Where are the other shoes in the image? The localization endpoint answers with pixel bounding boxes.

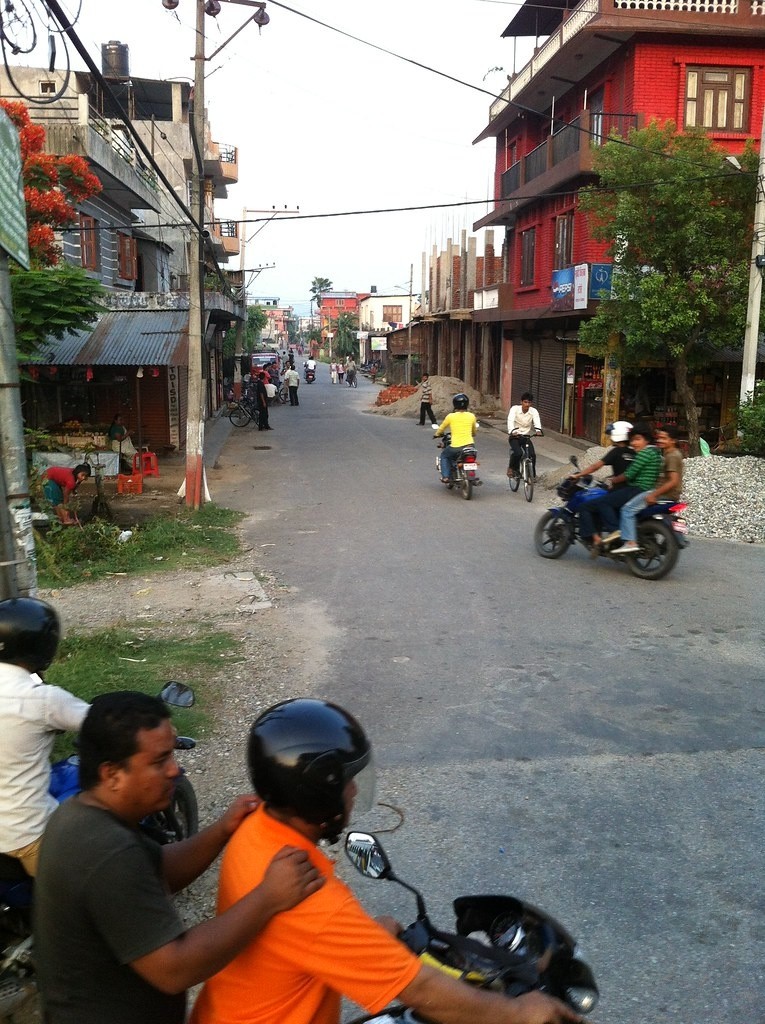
[590,538,602,559]
[610,542,642,553]
[291,403,295,406]
[295,402,299,406]
[63,519,79,525]
[529,475,537,483]
[416,422,424,426]
[265,425,274,430]
[602,531,620,543]
[507,467,514,478]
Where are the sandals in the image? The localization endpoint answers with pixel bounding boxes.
[439,476,449,483]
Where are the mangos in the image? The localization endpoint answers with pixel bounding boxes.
[64,420,80,426]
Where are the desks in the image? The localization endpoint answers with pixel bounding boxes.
[32,449,120,477]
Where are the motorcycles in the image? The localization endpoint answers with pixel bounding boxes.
[431,421,485,501]
[533,455,691,580]
[0,679,199,1022]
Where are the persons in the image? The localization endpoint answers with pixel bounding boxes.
[304,356,317,381]
[345,354,356,387]
[108,412,141,469]
[369,364,377,384]
[0,597,91,880]
[506,391,543,483]
[337,358,346,383]
[256,372,275,431]
[330,358,339,384]
[41,463,92,525]
[185,699,585,1024]
[298,345,305,357]
[271,348,294,369]
[568,422,684,554]
[433,393,477,482]
[32,690,328,1024]
[261,360,300,406]
[415,374,437,426]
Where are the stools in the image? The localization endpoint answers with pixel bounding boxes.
[132,443,150,452]
[133,452,159,478]
[116,474,143,494]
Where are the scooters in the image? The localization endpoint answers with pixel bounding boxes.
[345,370,359,387]
[345,829,604,1024]
[303,362,318,383]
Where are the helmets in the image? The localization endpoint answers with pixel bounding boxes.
[605,420,634,442]
[309,356,314,359]
[0,597,60,672]
[453,394,469,409]
[247,697,375,829]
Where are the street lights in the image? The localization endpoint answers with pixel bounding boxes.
[163,0,270,517]
[395,286,412,386]
[322,305,333,358]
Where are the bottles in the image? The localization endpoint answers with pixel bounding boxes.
[655,406,677,425]
[585,364,604,379]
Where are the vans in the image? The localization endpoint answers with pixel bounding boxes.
[251,353,280,381]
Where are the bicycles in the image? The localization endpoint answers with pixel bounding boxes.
[229,388,266,429]
[508,425,545,502]
[272,381,291,403]
